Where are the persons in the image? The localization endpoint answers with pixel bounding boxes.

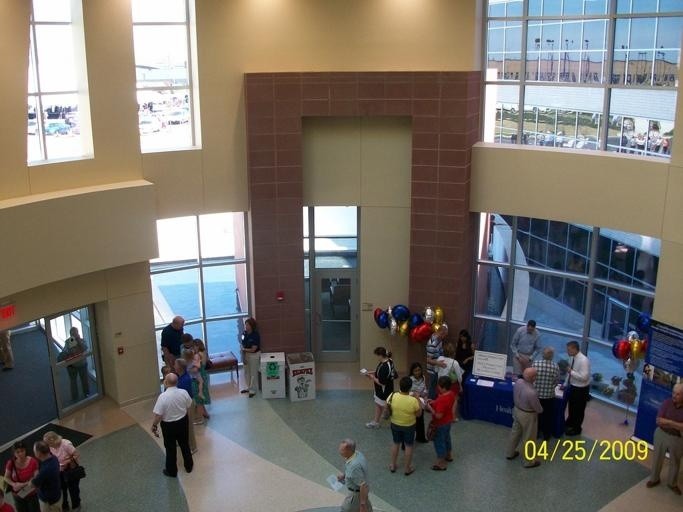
[646,384,683,497]
[149,316,211,477]
[360,326,477,474]
[508,319,590,469]
[54,327,89,400]
[336,439,374,511]
[1,430,81,512]
[236,317,260,396]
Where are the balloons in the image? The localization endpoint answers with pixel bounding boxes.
[371,303,449,343]
[613,330,647,422]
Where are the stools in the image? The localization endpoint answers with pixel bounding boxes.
[208,351,239,385]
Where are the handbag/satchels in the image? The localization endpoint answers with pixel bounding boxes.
[205,351,213,371]
[449,360,458,381]
[56,348,72,368]
[63,458,86,484]
[383,392,395,419]
[393,361,399,379]
[427,411,438,441]
[3,459,15,494]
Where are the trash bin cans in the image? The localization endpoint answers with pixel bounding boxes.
[260,352,286,399]
[287,352,315,401]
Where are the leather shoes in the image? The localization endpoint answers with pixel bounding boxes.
[524,460,540,468]
[249,393,254,398]
[241,390,249,393]
[505,451,519,460]
[647,479,660,488]
[163,469,177,478]
[667,484,681,495]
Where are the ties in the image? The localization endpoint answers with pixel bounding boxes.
[568,357,575,387]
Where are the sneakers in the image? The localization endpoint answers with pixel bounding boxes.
[191,449,197,455]
[193,421,204,425]
[366,421,380,429]
[415,439,429,443]
[71,506,81,512]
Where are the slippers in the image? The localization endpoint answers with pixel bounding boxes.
[430,464,448,472]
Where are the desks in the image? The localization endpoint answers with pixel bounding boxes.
[459,372,570,439]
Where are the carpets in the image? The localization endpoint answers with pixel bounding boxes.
[0,423,93,494]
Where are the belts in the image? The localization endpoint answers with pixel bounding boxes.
[660,427,681,437]
[349,488,360,492]
[571,386,590,390]
[515,406,536,413]
[519,351,533,355]
